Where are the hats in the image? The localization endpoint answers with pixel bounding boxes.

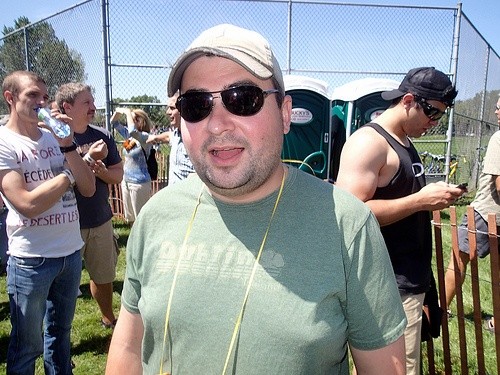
[381,67,458,108]
[166,24,285,99]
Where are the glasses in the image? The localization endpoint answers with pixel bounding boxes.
[174,83,279,123]
[414,95,445,121]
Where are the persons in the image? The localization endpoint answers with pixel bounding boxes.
[0,69,125,375]
[336,66,470,375]
[104,23,408,375]
[110,91,192,226]
[438,96,500,329]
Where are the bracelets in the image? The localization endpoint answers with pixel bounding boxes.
[84,153,96,166]
[62,169,76,187]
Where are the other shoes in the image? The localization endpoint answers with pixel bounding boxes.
[101,316,117,331]
[438,298,452,322]
[488,317,495,332]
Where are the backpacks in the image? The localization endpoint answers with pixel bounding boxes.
[141,145,158,181]
